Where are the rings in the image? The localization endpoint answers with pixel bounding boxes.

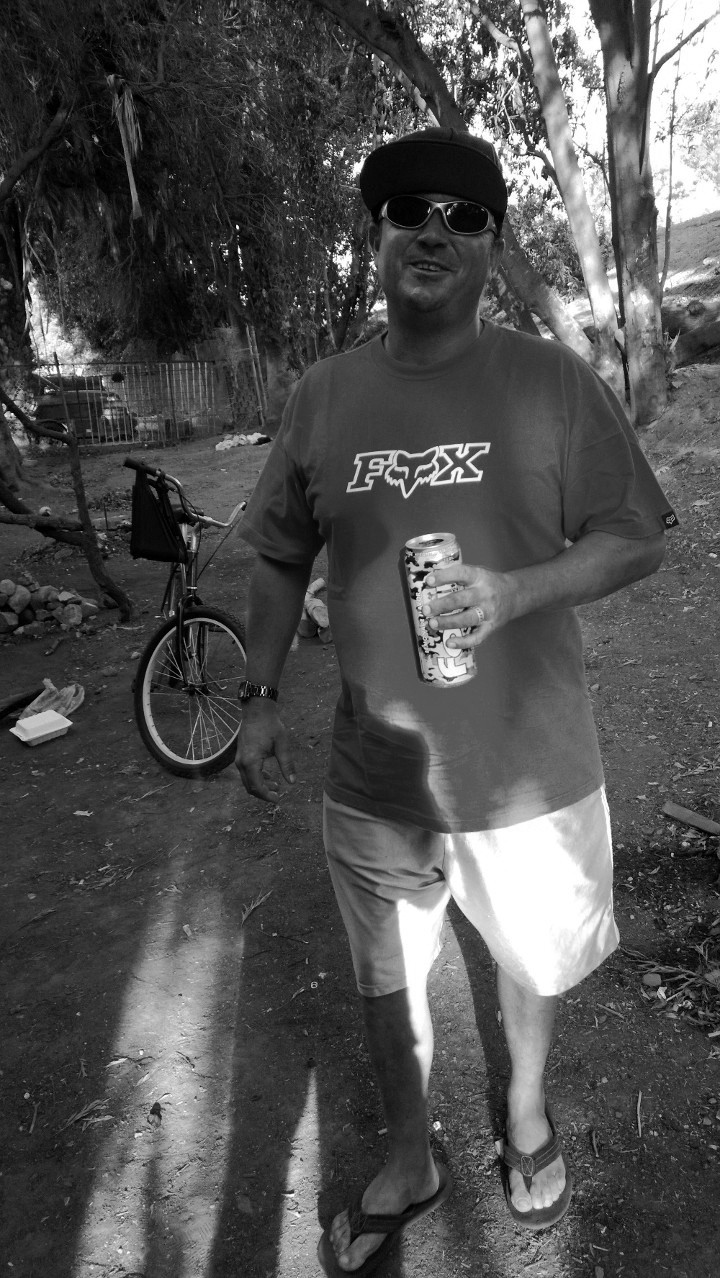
[470,606,485,626]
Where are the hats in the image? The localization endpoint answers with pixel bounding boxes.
[359,129,508,227]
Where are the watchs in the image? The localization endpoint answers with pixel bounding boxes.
[238,680,277,704]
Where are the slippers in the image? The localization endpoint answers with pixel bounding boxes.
[317,1164,453,1278]
[500,1097,573,1231]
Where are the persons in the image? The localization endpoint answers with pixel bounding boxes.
[245,126,683,1278]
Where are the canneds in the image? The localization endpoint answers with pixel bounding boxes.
[405,533,476,688]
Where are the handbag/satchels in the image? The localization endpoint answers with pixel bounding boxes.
[130,465,190,562]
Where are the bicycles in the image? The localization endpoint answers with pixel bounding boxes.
[123,453,260,780]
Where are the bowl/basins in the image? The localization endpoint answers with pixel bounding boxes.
[9,710,73,746]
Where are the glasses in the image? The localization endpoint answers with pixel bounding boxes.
[377,195,498,238]
